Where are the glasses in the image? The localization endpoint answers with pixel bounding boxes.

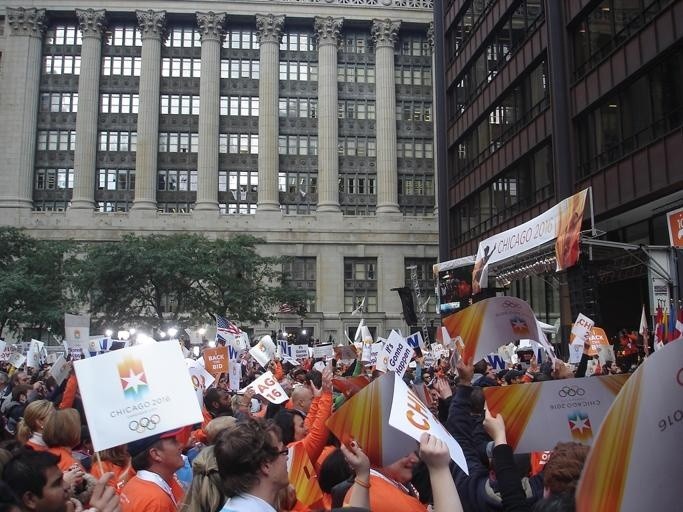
[276,448,289,455]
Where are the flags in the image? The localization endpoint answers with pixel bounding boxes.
[215,314,237,333]
[640,300,681,351]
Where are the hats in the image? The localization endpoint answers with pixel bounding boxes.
[127,429,183,456]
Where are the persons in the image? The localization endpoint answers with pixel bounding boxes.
[0,331,682,511]
[439,271,471,303]
[471,244,496,293]
